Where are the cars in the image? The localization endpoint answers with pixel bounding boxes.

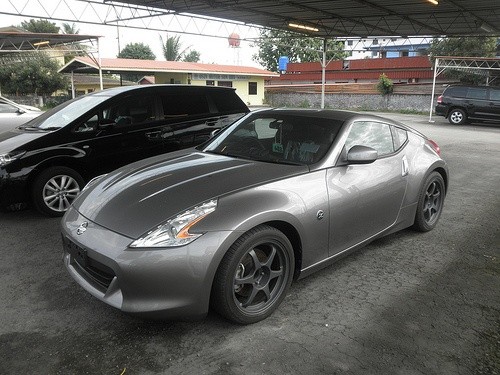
[0,95,47,135]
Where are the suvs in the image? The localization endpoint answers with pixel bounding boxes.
[434,83,500,126]
[0,81,259,219]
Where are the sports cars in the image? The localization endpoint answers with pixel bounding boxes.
[59,106,451,326]
